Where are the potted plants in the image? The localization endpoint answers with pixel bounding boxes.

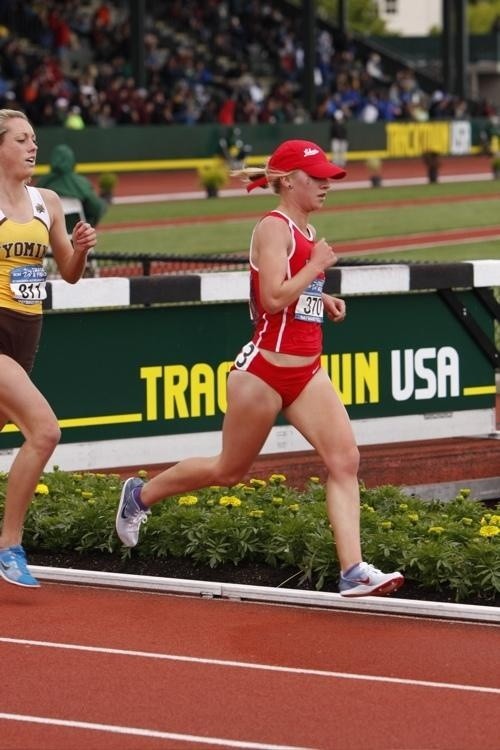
[199,165,228,196]
[98,172,117,203]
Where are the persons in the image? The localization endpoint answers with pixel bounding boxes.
[329,110,350,167]
[0,110,99,590]
[114,136,407,599]
[35,143,108,279]
[218,126,252,171]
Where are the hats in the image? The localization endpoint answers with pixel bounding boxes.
[264,138,348,181]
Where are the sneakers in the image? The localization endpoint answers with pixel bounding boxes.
[337,560,406,598]
[113,473,153,549]
[0,542,41,589]
